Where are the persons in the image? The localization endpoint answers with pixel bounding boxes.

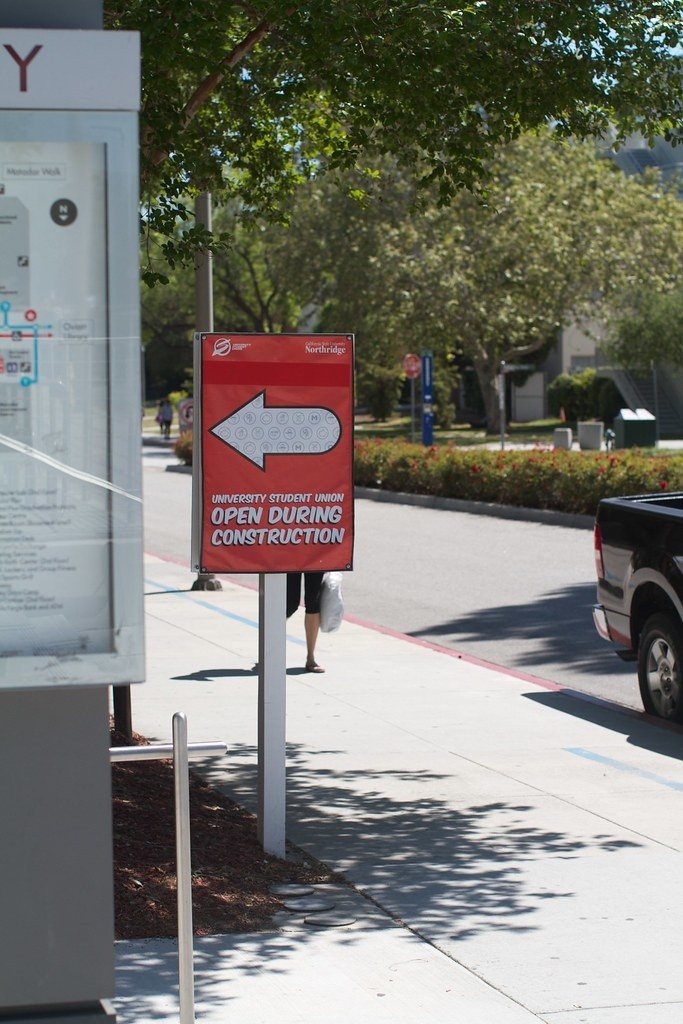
[286,571,327,674]
[155,398,173,440]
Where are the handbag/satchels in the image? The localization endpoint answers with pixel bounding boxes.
[319,570,344,632]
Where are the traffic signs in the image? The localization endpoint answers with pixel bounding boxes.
[191,330,356,578]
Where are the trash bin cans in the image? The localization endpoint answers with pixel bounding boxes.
[615,408,656,451]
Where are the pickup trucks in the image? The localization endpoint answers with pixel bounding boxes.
[590,490,683,726]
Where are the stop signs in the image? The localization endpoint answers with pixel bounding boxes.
[404,355,421,379]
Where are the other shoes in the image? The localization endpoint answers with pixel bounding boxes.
[306,662,324,672]
[252,662,259,672]
